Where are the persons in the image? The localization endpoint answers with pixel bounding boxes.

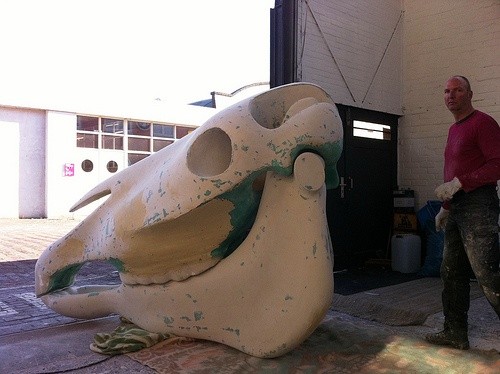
[426,76,500,350]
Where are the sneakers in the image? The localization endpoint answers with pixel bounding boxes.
[424,329,469,351]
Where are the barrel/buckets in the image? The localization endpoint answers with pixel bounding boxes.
[391,233,421,274]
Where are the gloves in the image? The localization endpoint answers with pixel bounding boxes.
[434,206,452,233]
[432,176,463,203]
